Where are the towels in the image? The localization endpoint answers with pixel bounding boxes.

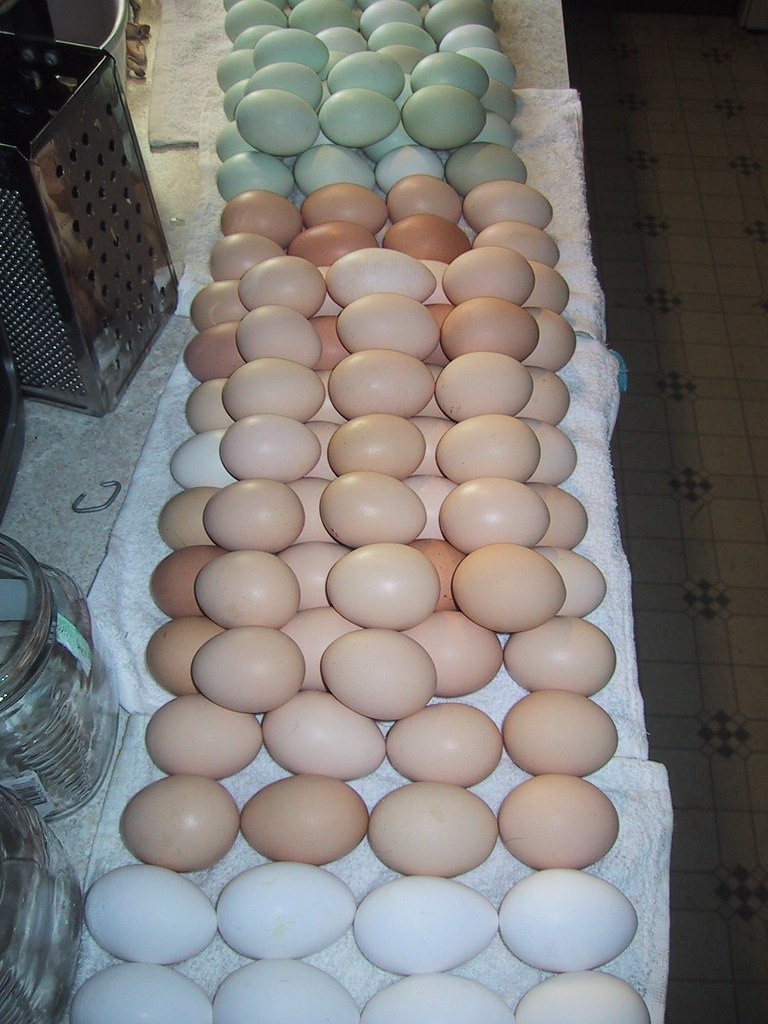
[57,2,677,1024]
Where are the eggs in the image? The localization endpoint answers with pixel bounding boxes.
[68,0,651,1024]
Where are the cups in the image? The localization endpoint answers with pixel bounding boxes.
[0,0,128,94]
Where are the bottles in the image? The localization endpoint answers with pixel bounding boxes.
[0,785,83,1024]
[0,533,118,821]
